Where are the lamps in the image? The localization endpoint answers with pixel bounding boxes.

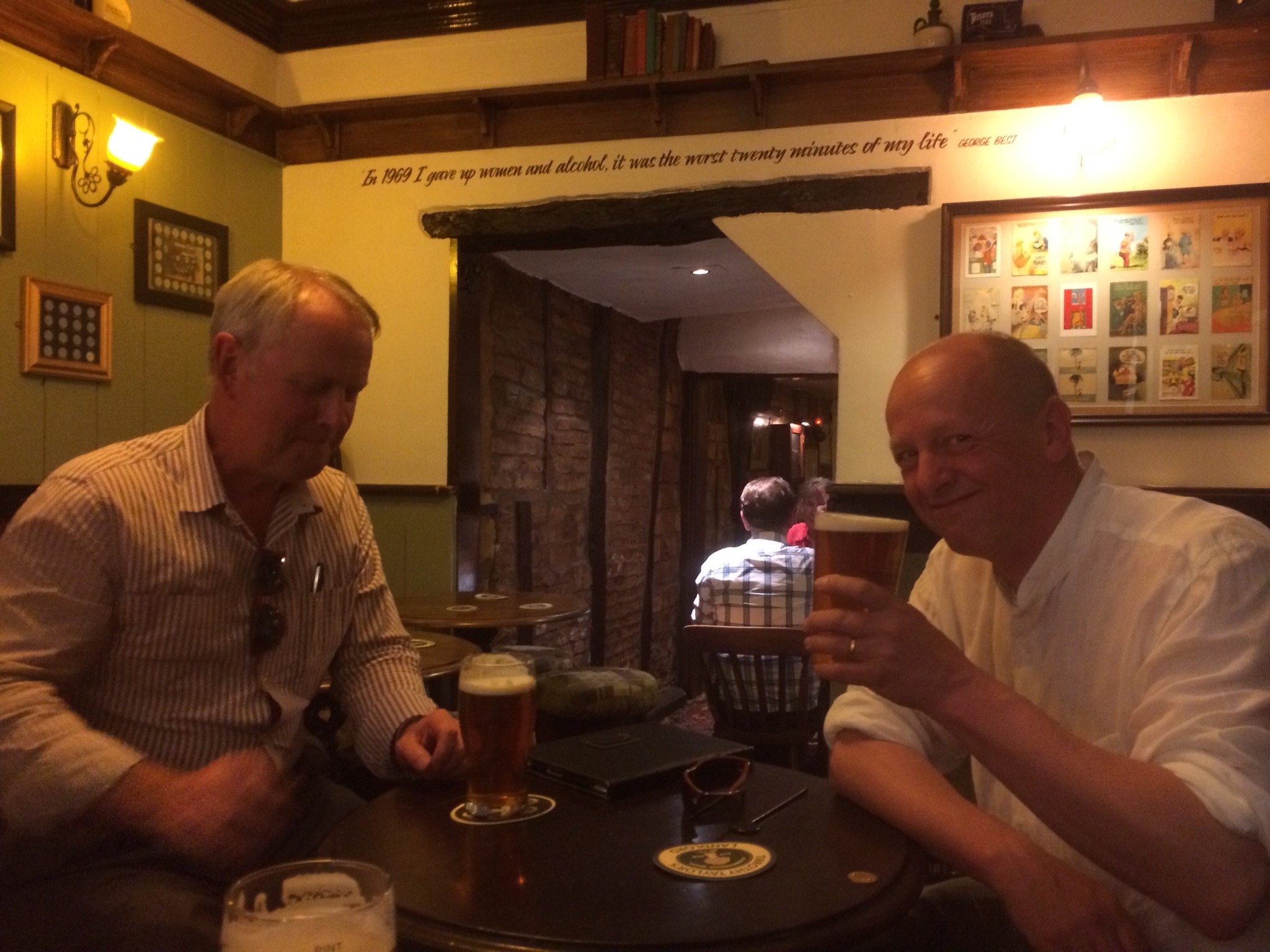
[1071,56,1105,105]
[51,100,168,211]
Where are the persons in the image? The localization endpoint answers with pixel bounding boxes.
[0,260,475,952]
[796,481,829,549]
[692,474,828,727]
[797,331,1270,952]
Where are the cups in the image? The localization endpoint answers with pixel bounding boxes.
[218,858,399,951]
[455,650,538,820]
[808,509,910,666]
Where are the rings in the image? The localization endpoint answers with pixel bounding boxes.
[849,638,856,655]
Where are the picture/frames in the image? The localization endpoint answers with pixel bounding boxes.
[17,275,115,384]
[131,198,229,313]
[0,98,18,254]
[935,182,1269,428]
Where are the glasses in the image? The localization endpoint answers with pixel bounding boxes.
[248,546,288,657]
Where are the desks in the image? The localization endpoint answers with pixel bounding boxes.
[304,633,482,762]
[682,624,830,773]
[390,591,588,652]
[316,758,925,952]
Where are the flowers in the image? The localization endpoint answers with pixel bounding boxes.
[786,523,809,548]
